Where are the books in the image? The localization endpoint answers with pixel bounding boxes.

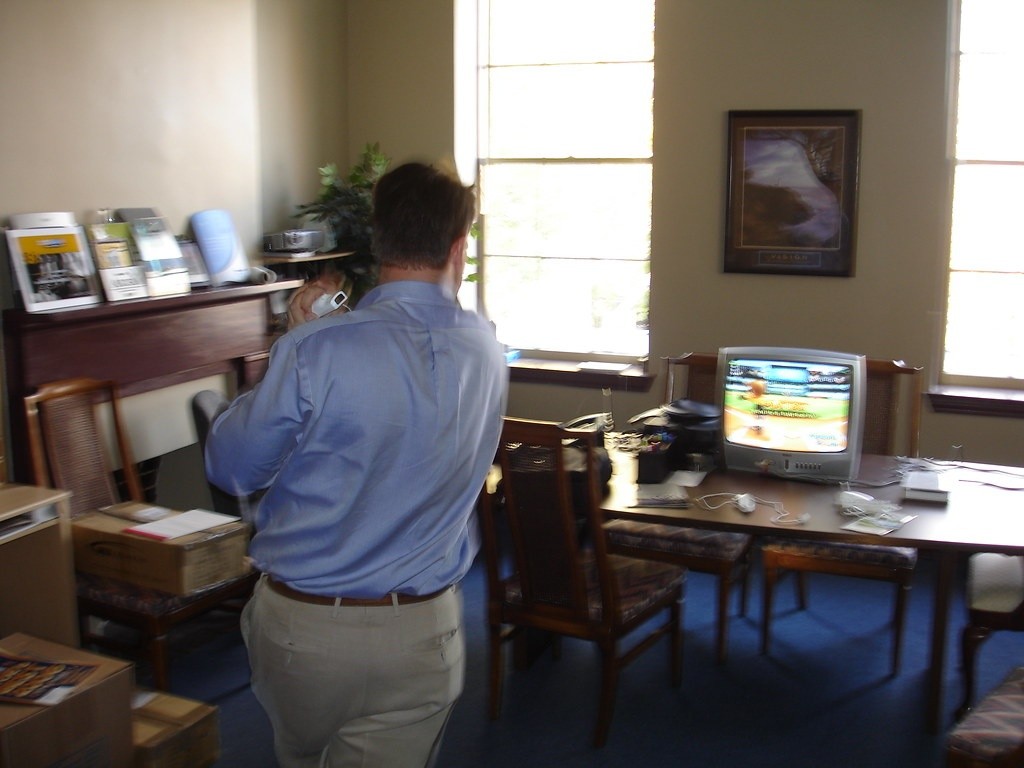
[906,470,951,503]
[627,483,690,508]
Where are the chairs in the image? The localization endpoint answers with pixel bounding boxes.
[952,551,1024,719]
[476,416,687,747]
[24,377,258,705]
[761,357,924,676]
[599,353,753,662]
[943,665,1024,768]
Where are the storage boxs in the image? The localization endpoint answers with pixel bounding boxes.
[132,685,223,768]
[0,632,133,768]
[70,500,251,598]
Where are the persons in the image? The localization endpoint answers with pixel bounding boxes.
[206,161,509,768]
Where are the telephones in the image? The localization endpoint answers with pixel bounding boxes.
[557,413,615,448]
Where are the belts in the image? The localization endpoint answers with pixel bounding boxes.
[266,574,454,606]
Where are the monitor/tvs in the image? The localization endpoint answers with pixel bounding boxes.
[713,347,867,483]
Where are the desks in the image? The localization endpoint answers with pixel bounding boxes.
[484,432,1024,552]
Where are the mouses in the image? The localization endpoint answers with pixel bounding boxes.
[734,494,757,513]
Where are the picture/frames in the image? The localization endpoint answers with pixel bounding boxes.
[5,226,103,312]
[722,108,862,281]
